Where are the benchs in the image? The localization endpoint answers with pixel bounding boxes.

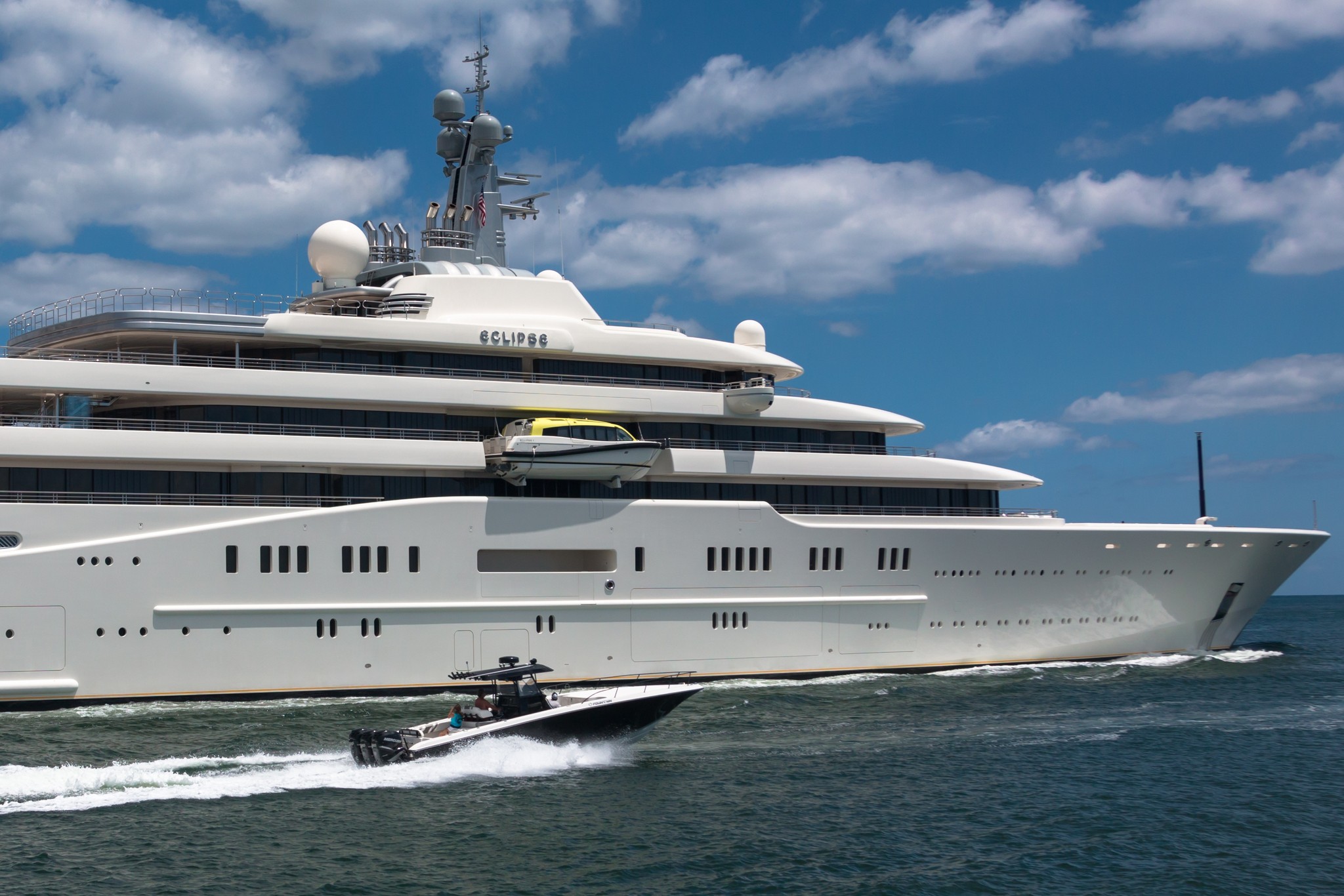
[446,706,498,735]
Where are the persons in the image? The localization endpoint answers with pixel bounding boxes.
[438,704,464,736]
[475,688,500,720]
[549,692,561,708]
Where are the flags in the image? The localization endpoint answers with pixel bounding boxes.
[477,182,486,228]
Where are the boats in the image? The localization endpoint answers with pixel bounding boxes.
[481,416,664,482]
[0,19,1331,708]
[349,656,705,768]
